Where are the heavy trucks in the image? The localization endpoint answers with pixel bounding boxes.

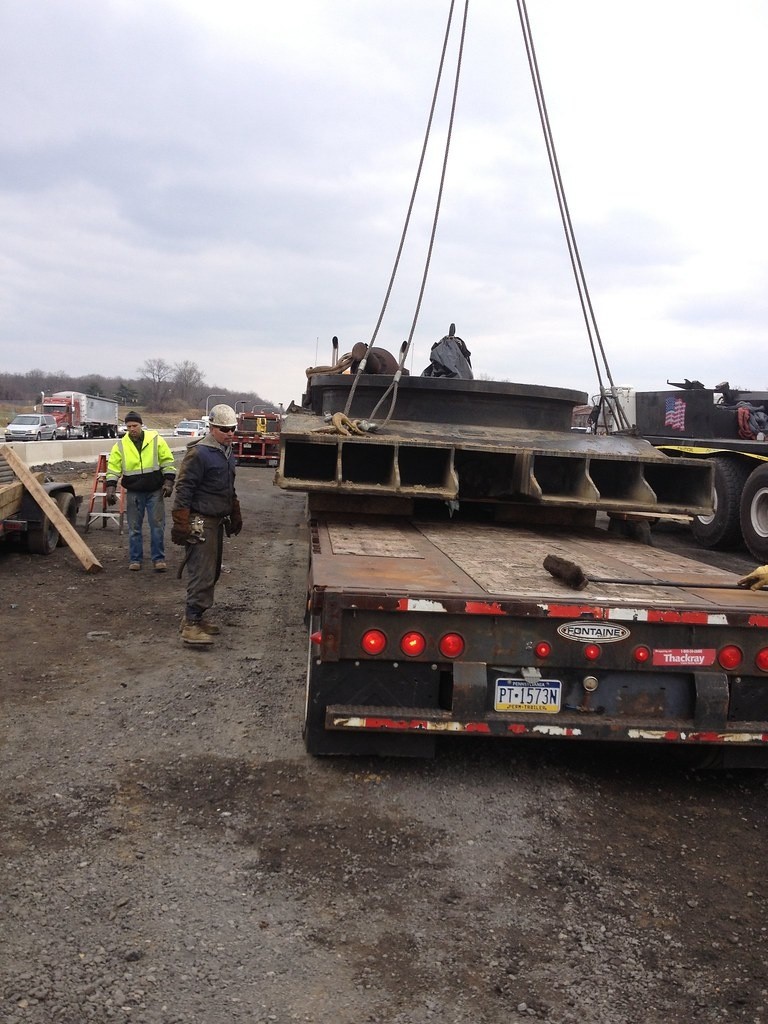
[273,369,767,760]
[40,390,119,440]
[575,384,768,566]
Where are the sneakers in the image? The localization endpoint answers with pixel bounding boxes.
[180,620,220,634]
[181,620,211,643]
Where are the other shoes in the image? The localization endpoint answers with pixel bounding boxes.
[129,563,140,570]
[154,561,167,569]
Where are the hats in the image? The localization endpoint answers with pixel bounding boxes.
[125,411,143,424]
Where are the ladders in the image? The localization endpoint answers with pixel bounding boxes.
[83,452,129,535]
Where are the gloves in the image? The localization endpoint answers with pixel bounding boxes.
[737,565,768,592]
[171,509,191,545]
[162,479,175,497]
[229,500,243,535]
[105,480,117,506]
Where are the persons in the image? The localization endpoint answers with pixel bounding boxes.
[106,411,176,573]
[737,564,768,593]
[170,403,243,645]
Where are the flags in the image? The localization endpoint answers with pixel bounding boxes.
[664,396,687,432]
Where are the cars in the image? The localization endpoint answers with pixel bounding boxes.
[192,420,211,436]
[173,420,207,437]
[118,422,148,438]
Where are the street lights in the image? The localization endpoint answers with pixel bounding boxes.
[235,401,251,414]
[252,404,266,414]
[206,395,226,416]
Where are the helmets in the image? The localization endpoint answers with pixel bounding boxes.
[208,404,239,427]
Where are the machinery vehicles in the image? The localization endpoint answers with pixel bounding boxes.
[230,411,282,468]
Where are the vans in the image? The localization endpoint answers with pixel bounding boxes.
[4,413,57,442]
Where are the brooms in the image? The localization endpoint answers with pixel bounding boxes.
[543,553,768,591]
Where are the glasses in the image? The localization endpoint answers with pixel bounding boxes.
[213,425,236,433]
